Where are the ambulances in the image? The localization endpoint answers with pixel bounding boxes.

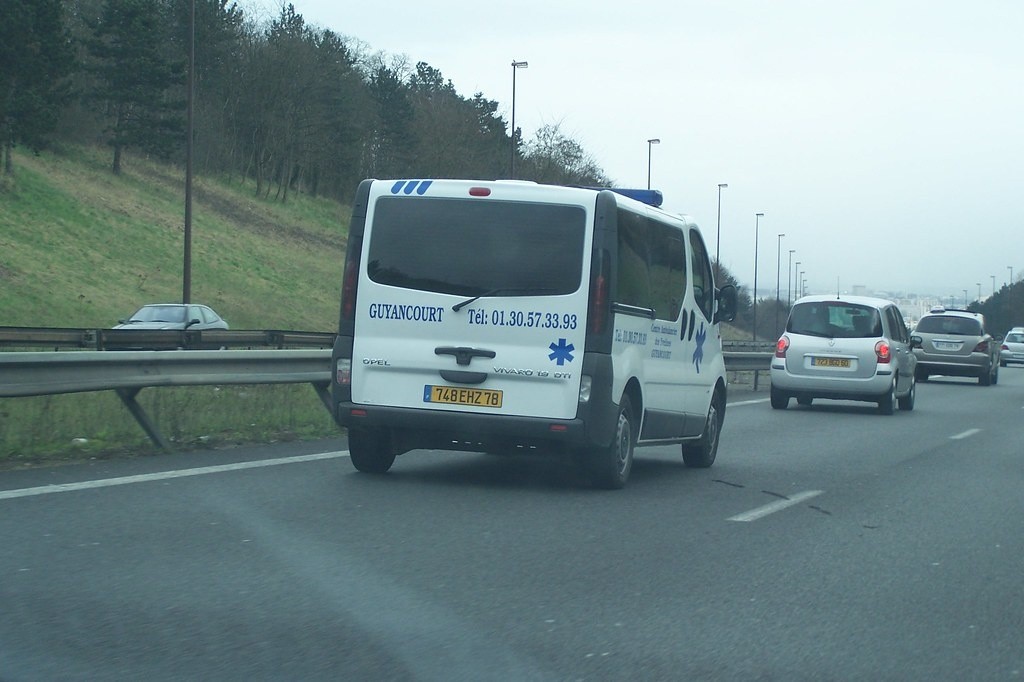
[331,177,740,492]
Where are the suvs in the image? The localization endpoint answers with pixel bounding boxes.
[910,305,1004,386]
[770,294,922,414]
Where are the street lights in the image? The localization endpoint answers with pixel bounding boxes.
[950,295,955,310]
[776,234,785,341]
[991,275,996,304]
[963,289,968,310]
[977,283,981,305]
[800,271,805,298]
[511,59,528,179]
[716,183,728,290]
[802,279,807,297]
[788,249,795,316]
[795,261,801,301]
[648,138,661,190]
[752,212,764,351]
[1007,266,1013,330]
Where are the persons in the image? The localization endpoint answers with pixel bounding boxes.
[816,306,830,324]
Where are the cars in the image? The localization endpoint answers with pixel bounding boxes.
[999,327,1024,368]
[106,303,231,351]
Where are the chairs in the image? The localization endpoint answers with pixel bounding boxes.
[853,316,872,334]
